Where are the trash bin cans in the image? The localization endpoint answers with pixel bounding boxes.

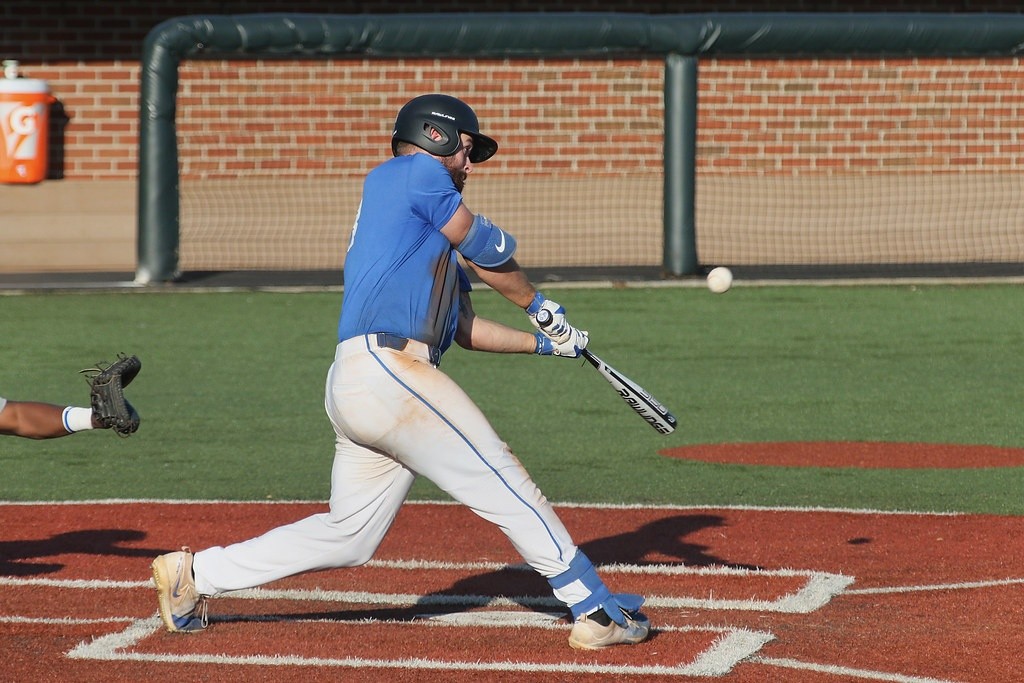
[0,76,58,186]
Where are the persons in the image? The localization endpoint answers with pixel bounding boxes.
[0,398,108,440]
[149,92,651,652]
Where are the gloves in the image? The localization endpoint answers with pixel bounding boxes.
[532,330,590,359]
[525,292,572,345]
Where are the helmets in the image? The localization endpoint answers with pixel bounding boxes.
[391,94,498,164]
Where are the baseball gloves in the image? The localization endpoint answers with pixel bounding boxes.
[78,353,142,439]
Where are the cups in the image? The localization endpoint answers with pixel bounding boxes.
[3,60,17,79]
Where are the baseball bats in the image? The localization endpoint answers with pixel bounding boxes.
[537,309,678,436]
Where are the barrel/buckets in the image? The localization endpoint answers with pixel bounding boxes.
[0,79,56,184]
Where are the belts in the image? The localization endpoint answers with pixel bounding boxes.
[378,334,441,367]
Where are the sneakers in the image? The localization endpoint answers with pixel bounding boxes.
[151,545,211,633]
[567,612,650,650]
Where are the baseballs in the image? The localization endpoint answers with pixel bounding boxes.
[705,265,735,295]
